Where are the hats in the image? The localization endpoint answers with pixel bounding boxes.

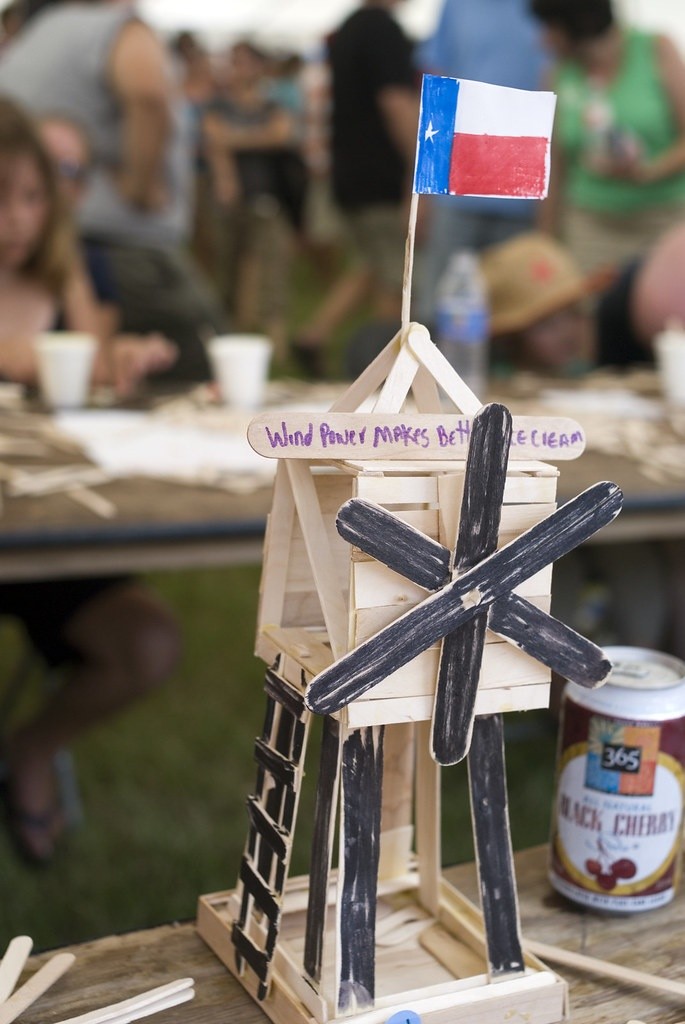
[481,231,592,333]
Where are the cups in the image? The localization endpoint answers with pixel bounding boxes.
[204,332,277,417]
[30,328,99,414]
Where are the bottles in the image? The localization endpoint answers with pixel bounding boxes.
[426,245,493,404]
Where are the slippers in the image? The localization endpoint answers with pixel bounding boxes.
[8,742,65,863]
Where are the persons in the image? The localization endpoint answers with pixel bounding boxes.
[294,0,420,379]
[0,0,226,380]
[415,0,555,321]
[0,95,226,867]
[506,0,685,265]
[483,222,685,657]
[173,34,309,239]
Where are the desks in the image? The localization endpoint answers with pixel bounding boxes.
[0,371,685,582]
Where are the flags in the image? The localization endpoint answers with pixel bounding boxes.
[411,72,557,201]
